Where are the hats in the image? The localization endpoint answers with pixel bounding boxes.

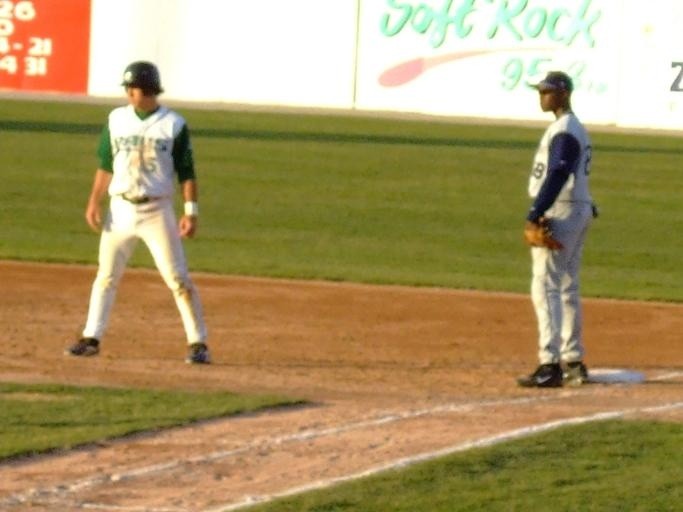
[529,71,574,93]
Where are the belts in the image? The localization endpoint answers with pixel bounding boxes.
[122,194,149,205]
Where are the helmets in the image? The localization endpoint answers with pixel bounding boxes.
[120,61,164,95]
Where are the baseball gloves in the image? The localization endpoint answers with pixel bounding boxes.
[524,217,564,250]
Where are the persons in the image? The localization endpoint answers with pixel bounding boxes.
[67,59,209,365]
[515,70,592,390]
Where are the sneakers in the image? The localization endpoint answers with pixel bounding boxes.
[63,337,100,358]
[516,364,563,388]
[185,343,210,365]
[565,361,588,385]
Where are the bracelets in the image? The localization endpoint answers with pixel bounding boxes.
[181,201,199,217]
